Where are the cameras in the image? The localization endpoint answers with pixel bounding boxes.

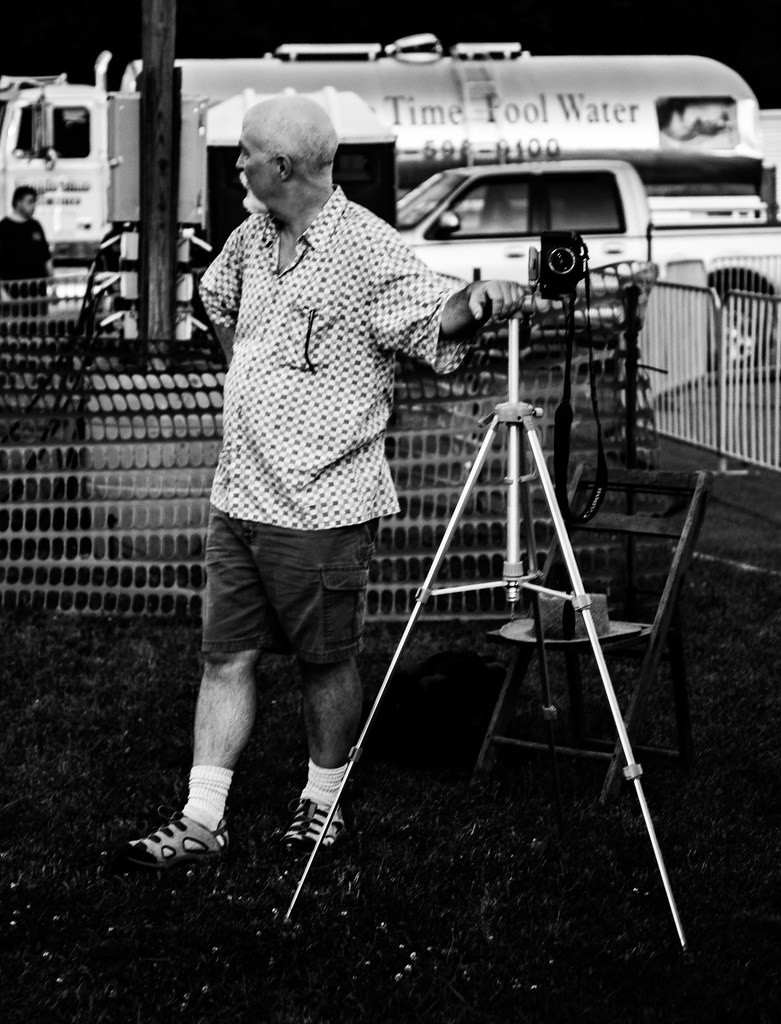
[528,231,586,304]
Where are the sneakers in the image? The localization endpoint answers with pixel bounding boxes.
[276,795,348,865]
[117,811,233,870]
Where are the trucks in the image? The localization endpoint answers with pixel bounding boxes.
[0,33,762,331]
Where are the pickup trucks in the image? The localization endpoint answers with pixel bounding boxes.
[393,157,780,371]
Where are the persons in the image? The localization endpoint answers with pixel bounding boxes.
[0,185,62,319]
[115,97,532,870]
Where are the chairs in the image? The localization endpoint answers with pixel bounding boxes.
[468,457,717,813]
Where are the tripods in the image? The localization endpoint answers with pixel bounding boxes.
[281,303,695,955]
